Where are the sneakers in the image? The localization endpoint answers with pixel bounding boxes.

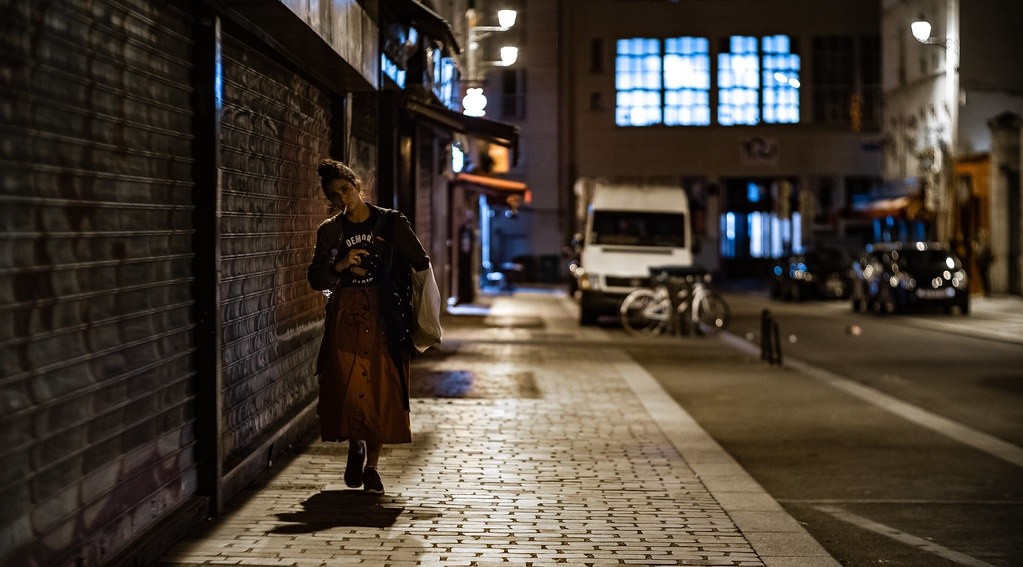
[363,463,385,494]
[344,443,365,488]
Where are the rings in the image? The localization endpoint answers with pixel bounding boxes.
[355,255,359,260]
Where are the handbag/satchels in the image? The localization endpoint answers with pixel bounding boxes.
[380,261,442,367]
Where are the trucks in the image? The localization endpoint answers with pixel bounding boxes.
[573,183,704,326]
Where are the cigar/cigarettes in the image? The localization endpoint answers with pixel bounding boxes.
[343,204,348,214]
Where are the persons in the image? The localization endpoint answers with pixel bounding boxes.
[306,161,429,495]
[972,227,994,299]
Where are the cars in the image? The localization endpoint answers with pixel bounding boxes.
[855,241,971,314]
[769,246,855,303]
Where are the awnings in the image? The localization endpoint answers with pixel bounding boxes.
[402,99,520,168]
[384,0,463,55]
[451,173,532,207]
[848,195,925,222]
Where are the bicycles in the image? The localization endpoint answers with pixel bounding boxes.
[619,270,731,340]
